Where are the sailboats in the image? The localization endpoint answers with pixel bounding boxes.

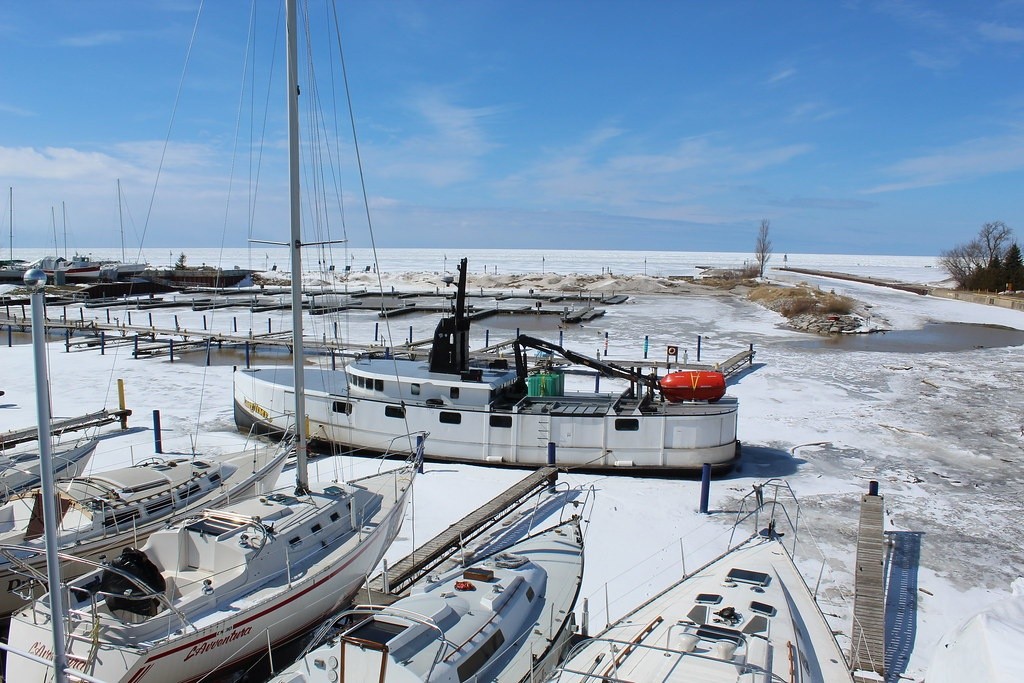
[0,0,432,683]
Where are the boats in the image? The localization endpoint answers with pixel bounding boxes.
[0,177,267,288]
[230,256,741,472]
[259,478,599,682]
[516,477,882,682]
[0,414,308,630]
[0,412,104,498]
